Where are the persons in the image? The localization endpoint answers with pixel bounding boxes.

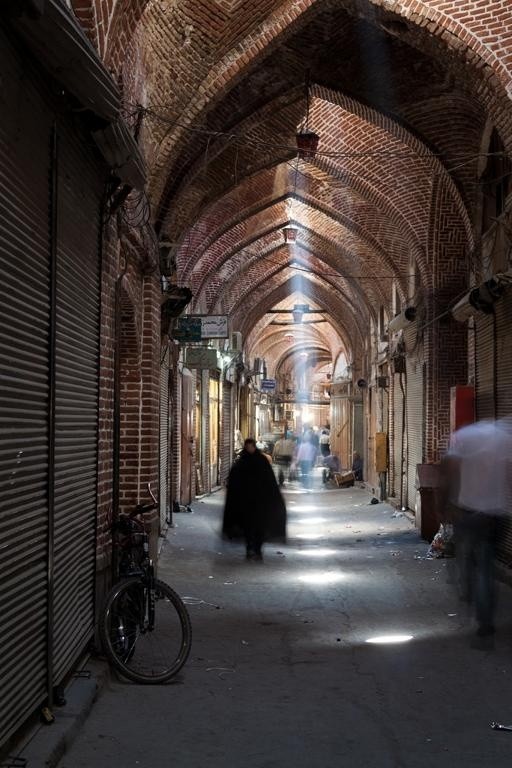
[233,420,364,488]
[222,436,285,561]
[438,387,512,653]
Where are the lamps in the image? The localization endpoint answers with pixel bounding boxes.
[295,84,321,159]
[293,311,303,323]
[282,227,299,244]
[448,290,484,325]
[385,306,416,334]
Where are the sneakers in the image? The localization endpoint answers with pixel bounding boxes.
[470,635,495,650]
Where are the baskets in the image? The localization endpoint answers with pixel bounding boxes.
[117,532,148,575]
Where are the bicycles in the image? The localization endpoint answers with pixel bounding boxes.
[98,480,198,687]
